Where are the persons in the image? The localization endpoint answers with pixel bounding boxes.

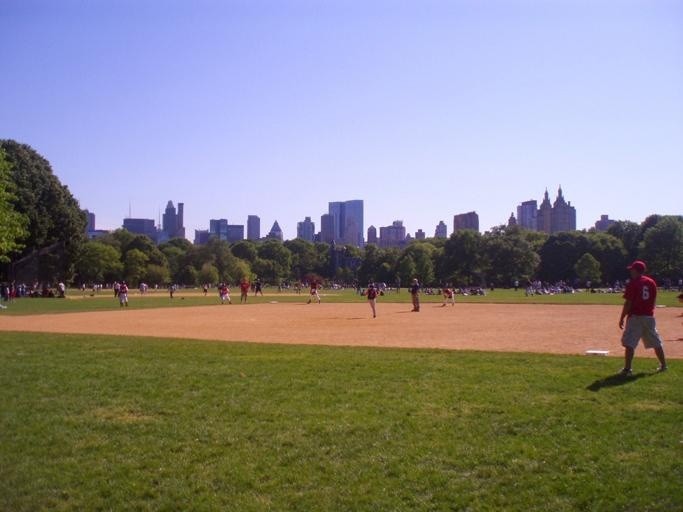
[140,281,148,295]
[169,283,179,298]
[442,289,454,306]
[423,281,485,296]
[279,278,342,303]
[617,261,667,377]
[118,281,128,307]
[352,279,387,297]
[585,279,629,294]
[202,278,263,304]
[677,294,683,341]
[1,278,65,303]
[515,280,519,291]
[82,280,121,297]
[366,285,377,318]
[408,279,420,312]
[525,279,575,297]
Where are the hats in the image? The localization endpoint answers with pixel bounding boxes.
[627,260,645,273]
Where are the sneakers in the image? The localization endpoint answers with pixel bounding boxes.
[619,368,632,376]
[657,366,668,371]
